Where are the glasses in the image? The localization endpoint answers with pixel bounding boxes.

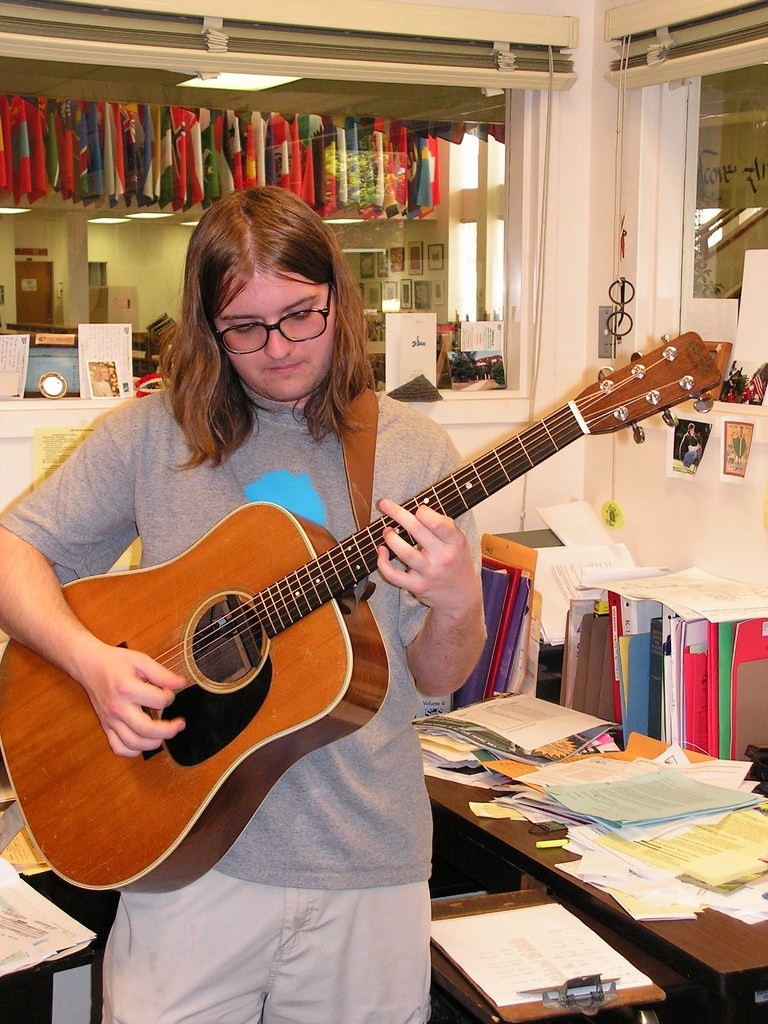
[210,282,332,354]
[606,277,635,345]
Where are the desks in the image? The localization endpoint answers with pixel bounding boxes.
[417,770,768,1024]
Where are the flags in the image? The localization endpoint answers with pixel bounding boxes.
[0,93,506,221]
[753,375,765,400]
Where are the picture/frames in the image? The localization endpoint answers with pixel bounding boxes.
[698,340,734,400]
[354,240,444,315]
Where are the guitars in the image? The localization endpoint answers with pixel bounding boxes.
[1,332,730,891]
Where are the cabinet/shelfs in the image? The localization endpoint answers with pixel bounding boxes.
[90,286,139,333]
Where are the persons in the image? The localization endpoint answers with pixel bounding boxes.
[678,361,759,473]
[92,363,113,397]
[0,185,488,1024]
